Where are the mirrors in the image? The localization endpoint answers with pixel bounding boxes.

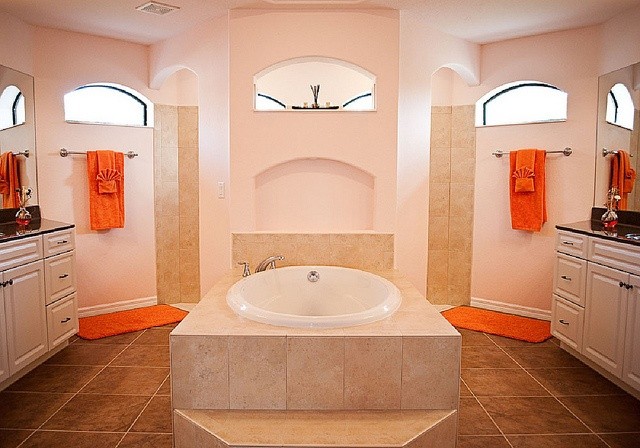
[591,62,640,213]
[0,64,40,210]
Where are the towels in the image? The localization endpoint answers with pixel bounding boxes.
[86,149,125,230]
[515,150,536,193]
[622,151,636,194]
[1,150,21,210]
[95,150,121,195]
[0,151,13,195]
[508,148,547,232]
[610,148,636,210]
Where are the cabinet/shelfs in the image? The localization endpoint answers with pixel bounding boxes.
[0,227,81,393]
[551,226,640,402]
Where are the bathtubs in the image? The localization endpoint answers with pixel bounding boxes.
[226,266,402,328]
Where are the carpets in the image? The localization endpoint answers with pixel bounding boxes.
[439,305,553,344]
[74,303,190,341]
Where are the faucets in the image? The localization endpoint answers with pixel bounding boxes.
[254,255,285,273]
